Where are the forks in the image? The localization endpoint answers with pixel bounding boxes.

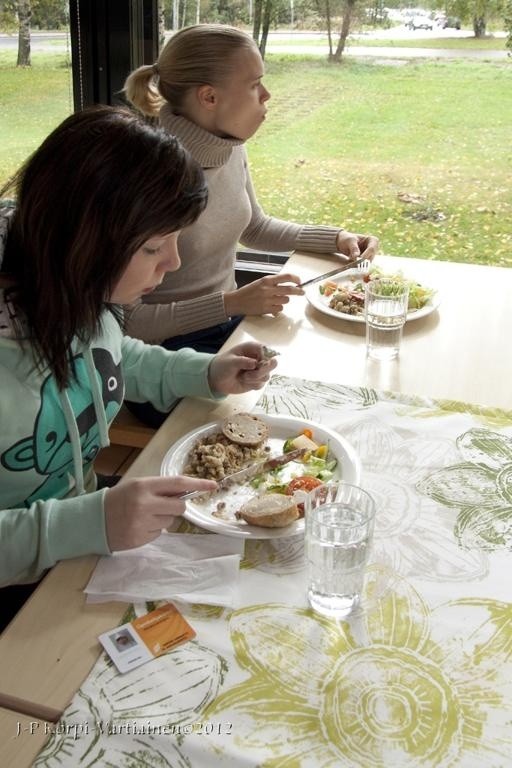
[250,343,279,370]
[355,256,370,272]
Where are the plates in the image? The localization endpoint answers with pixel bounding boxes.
[161,411,363,543]
[305,267,438,330]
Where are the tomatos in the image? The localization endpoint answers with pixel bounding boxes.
[286,476,324,508]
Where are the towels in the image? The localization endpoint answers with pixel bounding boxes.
[66,371,510,768]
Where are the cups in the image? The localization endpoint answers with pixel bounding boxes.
[359,281,410,359]
[301,482,376,618]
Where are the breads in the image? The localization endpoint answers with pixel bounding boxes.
[240,492,298,528]
[221,412,269,450]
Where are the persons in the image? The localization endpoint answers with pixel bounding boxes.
[115,632,136,652]
[0,104,278,632]
[115,23,381,429]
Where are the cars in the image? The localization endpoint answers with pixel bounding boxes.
[401,10,462,29]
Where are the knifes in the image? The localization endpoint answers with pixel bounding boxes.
[296,254,376,290]
[175,448,312,503]
